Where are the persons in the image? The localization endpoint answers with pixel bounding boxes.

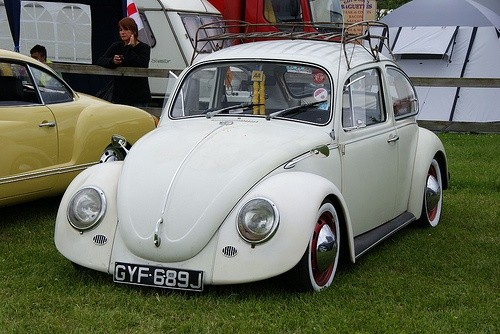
[97,16,152,109]
[29,44,54,87]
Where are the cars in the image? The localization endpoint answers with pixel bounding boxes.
[0,49,161,209]
[53,18,452,293]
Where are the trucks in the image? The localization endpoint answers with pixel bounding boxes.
[206,0,349,45]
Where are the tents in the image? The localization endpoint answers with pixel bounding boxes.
[362,0,500,135]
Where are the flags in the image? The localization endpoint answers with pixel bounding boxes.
[127,2,144,31]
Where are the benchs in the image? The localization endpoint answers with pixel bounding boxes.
[240,109,376,129]
[24,85,66,104]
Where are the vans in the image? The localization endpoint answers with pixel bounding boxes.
[0,0,232,99]
